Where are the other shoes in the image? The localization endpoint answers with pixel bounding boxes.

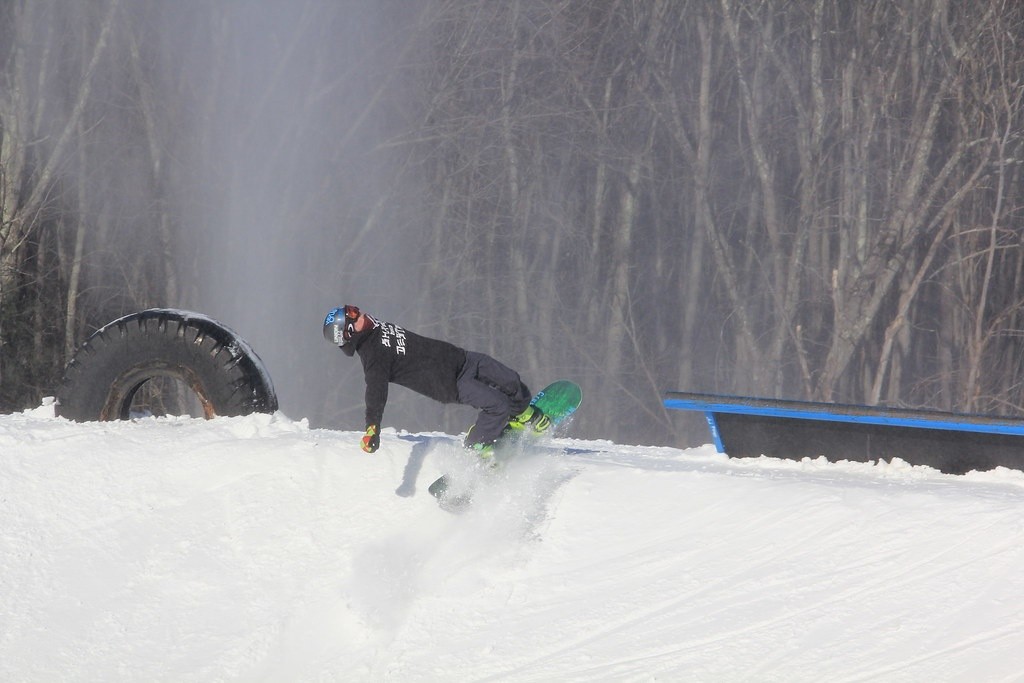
[513,408,551,436]
[471,445,499,468]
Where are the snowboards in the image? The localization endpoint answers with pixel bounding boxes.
[427,379,584,510]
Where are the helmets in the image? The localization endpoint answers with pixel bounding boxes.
[324,305,348,347]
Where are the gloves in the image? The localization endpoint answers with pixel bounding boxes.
[360,425,380,453]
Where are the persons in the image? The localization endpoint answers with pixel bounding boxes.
[322,304,553,471]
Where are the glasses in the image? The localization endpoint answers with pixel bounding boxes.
[349,305,359,321]
